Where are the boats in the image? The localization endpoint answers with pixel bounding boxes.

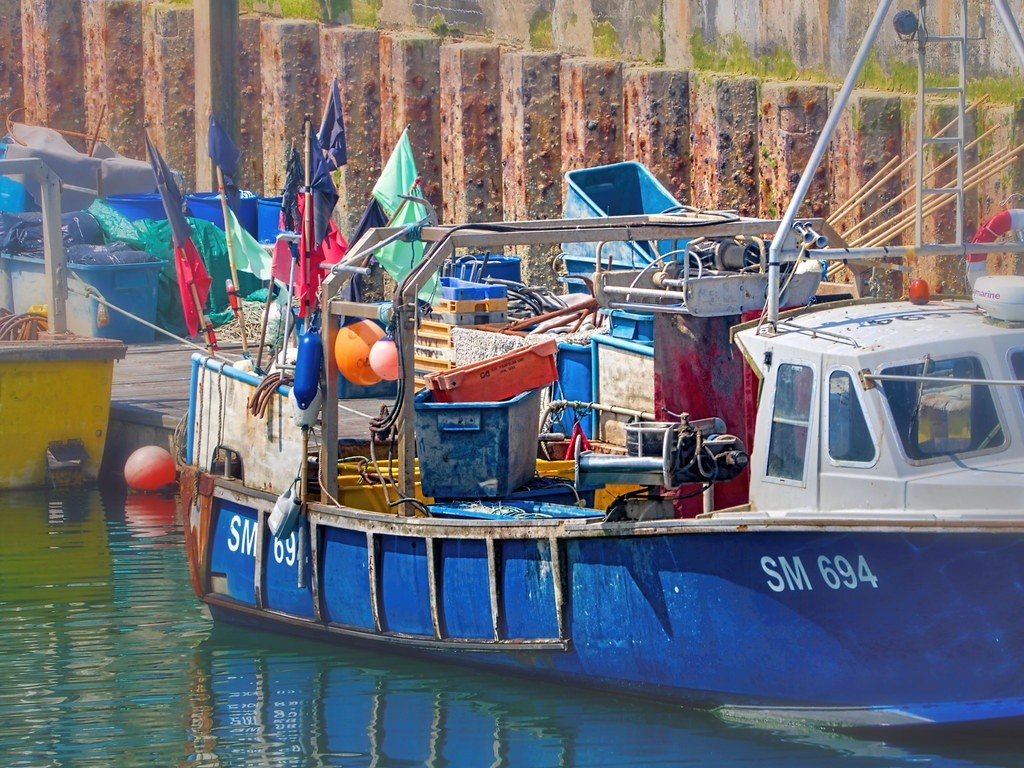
[0,157,127,491]
[179,0,1024,728]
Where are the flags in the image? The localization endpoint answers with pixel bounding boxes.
[145,79,444,339]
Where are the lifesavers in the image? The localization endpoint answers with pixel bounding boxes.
[966,208,1024,293]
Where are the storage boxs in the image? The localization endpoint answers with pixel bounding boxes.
[439,277,507,300]
[560,161,696,266]
[431,298,507,313]
[2,253,169,342]
[423,338,559,402]
[422,313,508,326]
[599,304,687,347]
[551,342,592,439]
[560,255,652,279]
[557,277,594,295]
[413,382,550,498]
